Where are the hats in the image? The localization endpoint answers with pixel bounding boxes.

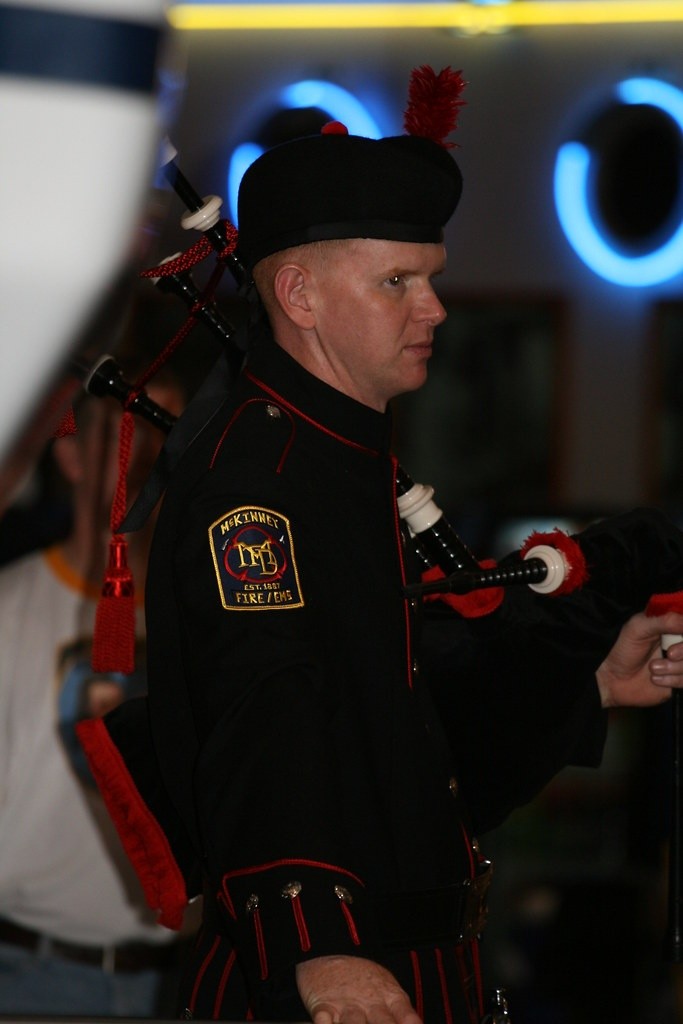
[230,118,464,265]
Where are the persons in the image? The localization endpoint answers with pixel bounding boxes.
[0,2,683,1023]
[52,64,683,1024]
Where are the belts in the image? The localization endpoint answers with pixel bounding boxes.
[0,920,168,974]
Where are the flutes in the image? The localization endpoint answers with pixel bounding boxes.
[79,128,683,612]
